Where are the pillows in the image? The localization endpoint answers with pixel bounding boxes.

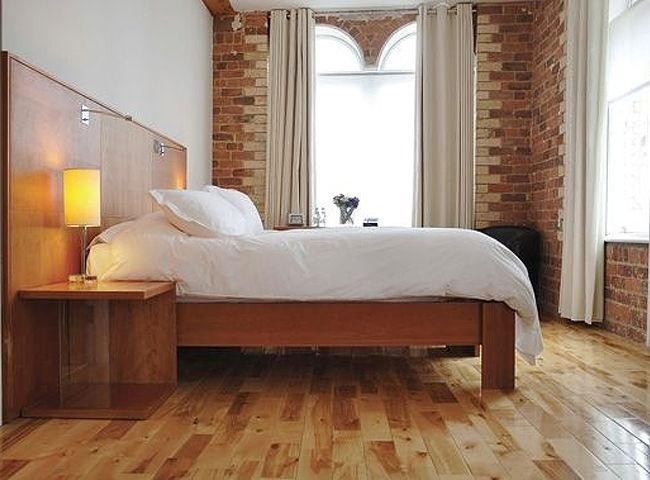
[201,183,264,231]
[147,188,261,238]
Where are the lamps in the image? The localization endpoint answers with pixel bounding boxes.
[61,168,103,282]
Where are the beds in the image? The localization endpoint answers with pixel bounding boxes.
[83,209,544,390]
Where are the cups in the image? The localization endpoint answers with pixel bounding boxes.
[84,259,98,283]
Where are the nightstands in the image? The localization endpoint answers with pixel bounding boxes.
[16,278,179,421]
